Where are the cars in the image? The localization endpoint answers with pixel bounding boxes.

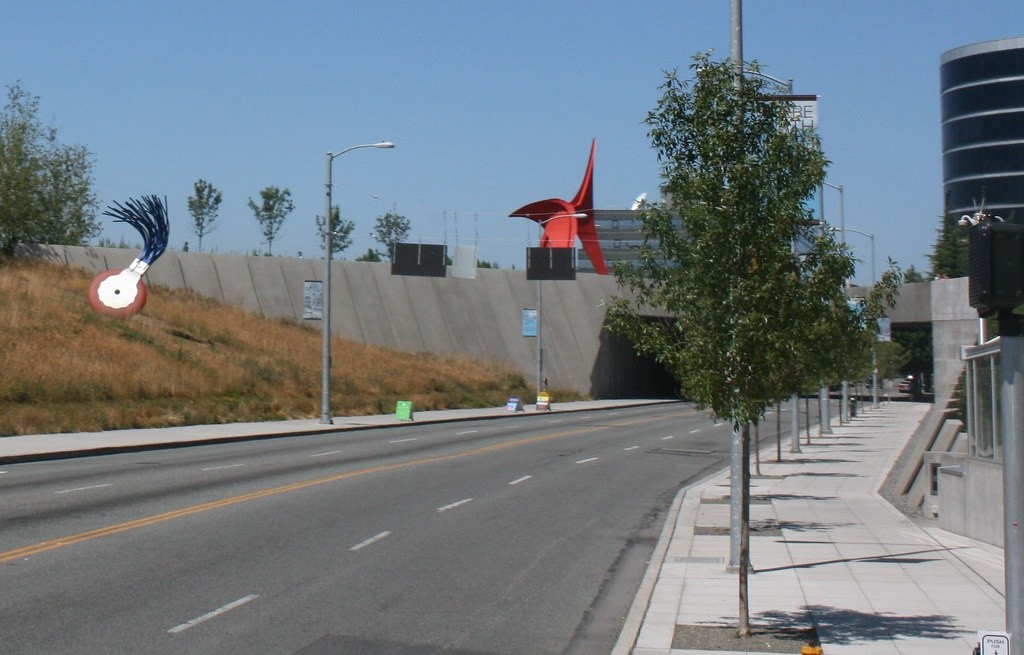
[898,380,912,393]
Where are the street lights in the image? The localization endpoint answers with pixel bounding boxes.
[536,211,588,410]
[802,175,851,434]
[829,226,884,410]
[320,138,397,427]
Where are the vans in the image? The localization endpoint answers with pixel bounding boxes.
[866,375,884,388]
